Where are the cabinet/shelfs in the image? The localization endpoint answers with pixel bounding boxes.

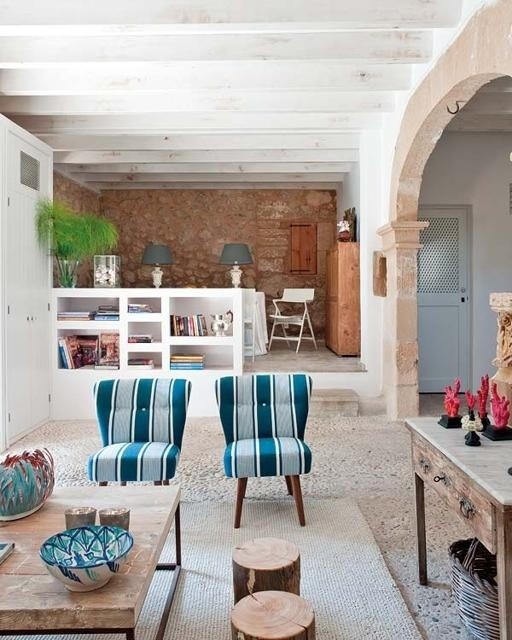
[49,288,242,419]
[324,241,359,355]
[4,190,49,440]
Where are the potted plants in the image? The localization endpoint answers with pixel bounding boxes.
[33,198,120,288]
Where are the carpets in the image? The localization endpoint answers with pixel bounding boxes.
[0,497,424,640]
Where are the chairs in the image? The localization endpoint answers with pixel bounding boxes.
[85,373,314,528]
[243,286,318,363]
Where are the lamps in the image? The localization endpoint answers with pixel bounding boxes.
[218,243,252,288]
[142,244,174,289]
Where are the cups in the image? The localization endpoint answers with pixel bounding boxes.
[64,508,130,532]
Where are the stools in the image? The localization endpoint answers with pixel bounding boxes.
[226,534,319,640]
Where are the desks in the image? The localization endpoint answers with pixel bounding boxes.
[403,416,511,639]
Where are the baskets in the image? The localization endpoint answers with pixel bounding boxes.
[447,537,499,640]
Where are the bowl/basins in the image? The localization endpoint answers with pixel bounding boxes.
[37,525,133,591]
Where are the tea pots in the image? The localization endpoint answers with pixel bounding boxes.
[210,311,233,338]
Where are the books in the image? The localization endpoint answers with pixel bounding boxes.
[57,303,208,369]
